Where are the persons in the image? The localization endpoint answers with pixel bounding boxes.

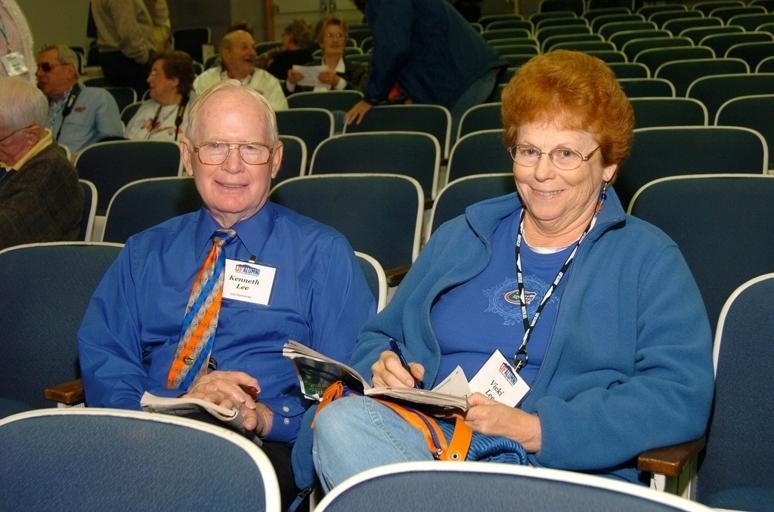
[341,1,501,131]
[125,51,197,142]
[191,30,289,112]
[0,0,37,86]
[266,16,365,97]
[313,47,716,497]
[34,43,124,160]
[91,0,171,92]
[74,74,377,495]
[1,77,83,248]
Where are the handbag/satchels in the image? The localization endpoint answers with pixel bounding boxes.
[310,380,528,466]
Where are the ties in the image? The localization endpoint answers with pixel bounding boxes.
[168,229,236,390]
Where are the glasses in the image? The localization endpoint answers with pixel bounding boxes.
[35,63,64,72]
[193,142,273,165]
[507,143,601,170]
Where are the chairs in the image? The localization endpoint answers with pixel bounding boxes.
[612,123,772,213]
[99,174,209,243]
[627,97,709,130]
[454,102,506,138]
[180,0,769,95]
[262,169,427,287]
[69,137,186,219]
[623,170,772,346]
[423,170,520,250]
[283,89,367,116]
[269,133,308,191]
[711,95,772,150]
[269,107,335,164]
[444,126,518,184]
[339,102,452,165]
[634,271,773,511]
[2,404,285,511]
[4,238,128,418]
[306,128,444,213]
[308,458,710,511]
[350,249,389,315]
[68,178,98,244]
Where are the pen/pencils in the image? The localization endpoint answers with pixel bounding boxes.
[238,384,258,396]
[388,337,425,389]
